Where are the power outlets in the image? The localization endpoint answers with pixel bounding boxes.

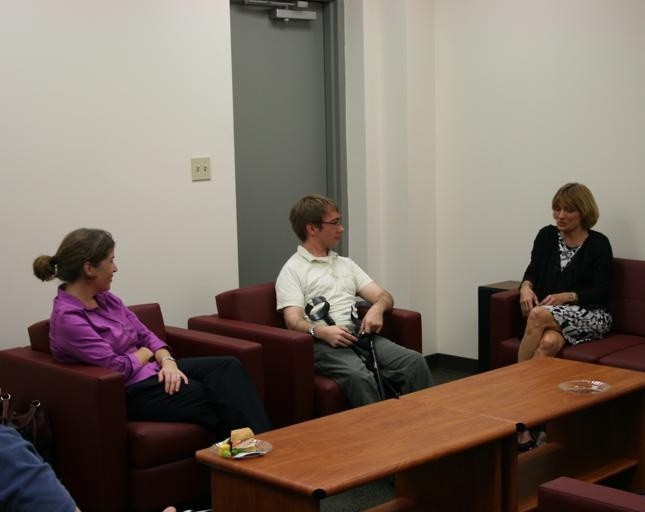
[192,157,211,181]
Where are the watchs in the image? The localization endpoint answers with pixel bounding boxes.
[308,323,322,337]
[160,355,176,365]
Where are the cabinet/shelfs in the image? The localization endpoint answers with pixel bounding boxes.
[478,281,520,374]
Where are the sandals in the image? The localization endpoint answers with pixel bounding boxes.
[518,430,535,450]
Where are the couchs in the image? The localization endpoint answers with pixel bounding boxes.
[537,476,645,512]
[489,258,645,452]
[0,303,264,512]
[187,283,423,426]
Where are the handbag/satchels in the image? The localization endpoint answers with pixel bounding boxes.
[1,393,54,468]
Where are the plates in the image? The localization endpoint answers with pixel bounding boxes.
[215,438,271,459]
[558,378,611,394]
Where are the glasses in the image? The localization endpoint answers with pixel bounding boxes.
[308,219,341,226]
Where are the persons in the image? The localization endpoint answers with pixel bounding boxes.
[0,422,194,512]
[277,195,438,408]
[33,229,274,436]
[518,183,618,453]
